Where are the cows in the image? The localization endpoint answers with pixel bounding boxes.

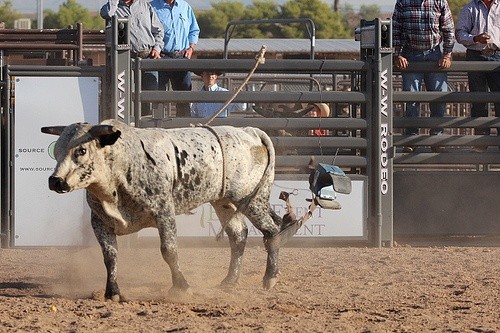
[41,118,294,302]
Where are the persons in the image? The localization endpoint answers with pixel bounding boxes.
[392,0,455,153]
[455,0,500,153]
[191,71,247,118]
[149,0,200,118]
[306,103,330,136]
[99,0,164,117]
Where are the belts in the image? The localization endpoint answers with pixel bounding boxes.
[163,52,184,57]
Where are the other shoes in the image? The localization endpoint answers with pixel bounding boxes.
[432,146,448,153]
[403,145,415,152]
[472,146,487,153]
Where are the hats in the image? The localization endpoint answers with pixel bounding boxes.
[302,102,330,118]
[194,71,223,76]
